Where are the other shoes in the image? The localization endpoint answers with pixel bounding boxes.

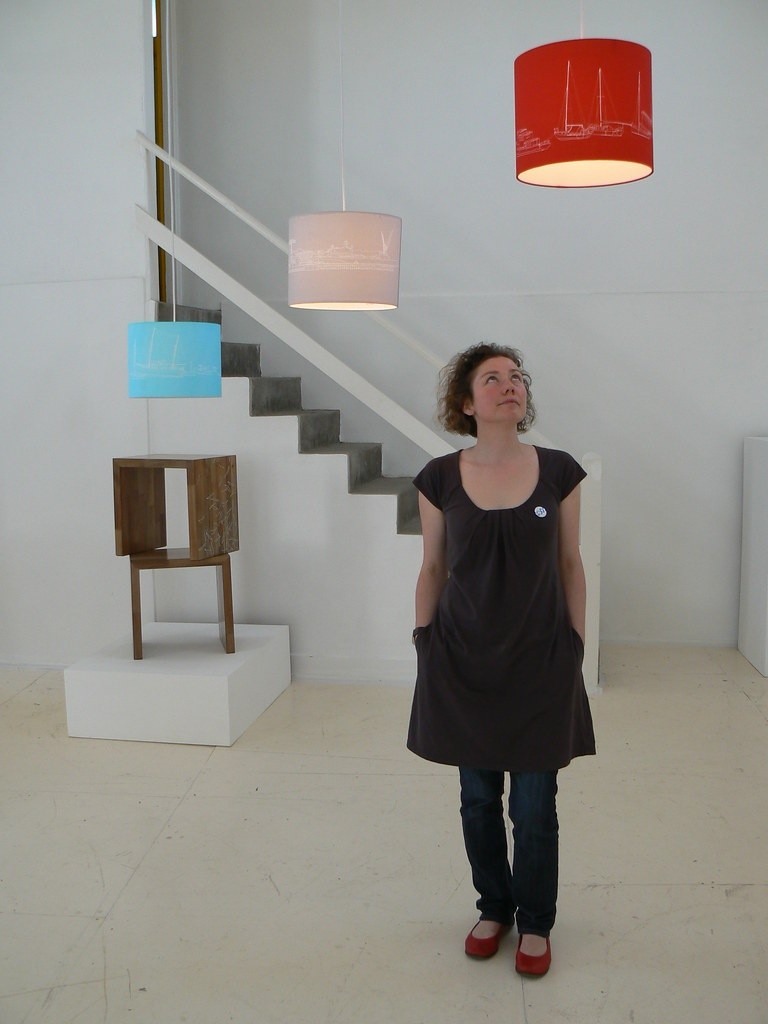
[465,919,511,958]
[515,932,552,977]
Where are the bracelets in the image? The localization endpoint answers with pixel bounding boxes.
[413,626,425,642]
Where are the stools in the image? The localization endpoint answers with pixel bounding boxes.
[129,548,235,660]
[113,453,239,561]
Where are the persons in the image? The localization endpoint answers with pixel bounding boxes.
[406,342,596,978]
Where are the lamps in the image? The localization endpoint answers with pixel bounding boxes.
[288,0,403,312]
[127,0,223,398]
[513,0,653,188]
